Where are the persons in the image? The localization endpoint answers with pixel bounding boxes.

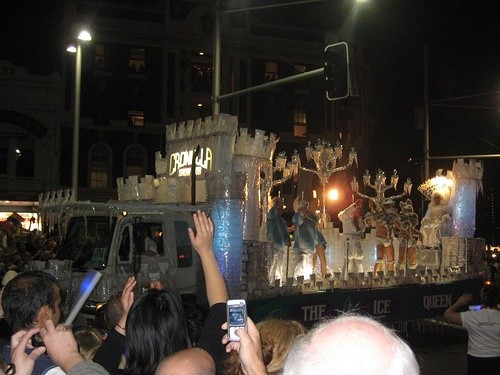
[288,196,320,290]
[372,207,398,278]
[422,193,450,248]
[396,204,421,277]
[338,199,364,281]
[267,197,291,288]
[0,217,110,375]
[444,285,500,375]
[96,209,422,375]
[289,197,328,286]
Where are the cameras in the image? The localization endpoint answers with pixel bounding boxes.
[31,332,46,348]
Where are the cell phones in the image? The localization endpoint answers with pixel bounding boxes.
[226,298,247,342]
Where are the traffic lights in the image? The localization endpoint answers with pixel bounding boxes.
[322,42,351,101]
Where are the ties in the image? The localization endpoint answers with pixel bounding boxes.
[353,215,361,232]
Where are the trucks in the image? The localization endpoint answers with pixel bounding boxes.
[46,146,485,349]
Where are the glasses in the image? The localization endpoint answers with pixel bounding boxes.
[5,363,15,375]
[112,291,121,298]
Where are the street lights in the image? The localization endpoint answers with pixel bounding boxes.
[65,27,92,202]
